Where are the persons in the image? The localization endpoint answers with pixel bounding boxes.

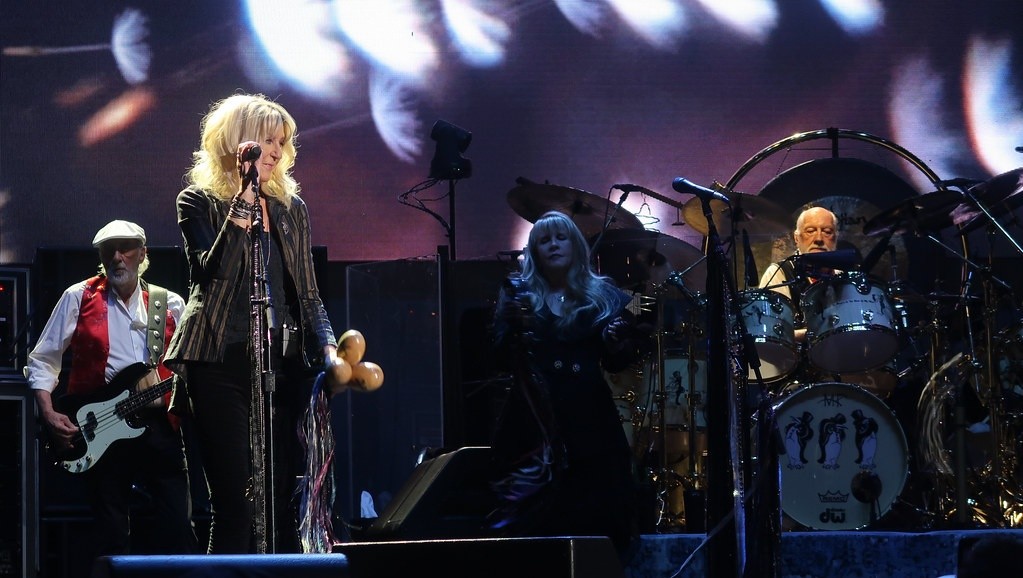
[23,221,201,556]
[747,207,896,389]
[480,210,634,538]
[161,93,338,555]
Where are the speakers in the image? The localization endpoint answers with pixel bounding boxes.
[90,553,352,578]
[0,378,40,578]
[333,536,626,578]
[363,445,498,541]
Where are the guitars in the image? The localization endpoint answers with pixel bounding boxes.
[36,362,175,479]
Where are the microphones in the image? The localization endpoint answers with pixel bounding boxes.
[858,224,899,273]
[944,178,982,186]
[742,229,759,287]
[673,177,729,204]
[616,183,681,209]
[246,147,261,161]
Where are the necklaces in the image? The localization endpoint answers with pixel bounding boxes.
[557,295,564,302]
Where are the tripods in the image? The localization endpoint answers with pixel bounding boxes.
[938,228,1023,530]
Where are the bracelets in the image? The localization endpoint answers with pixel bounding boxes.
[228,195,253,221]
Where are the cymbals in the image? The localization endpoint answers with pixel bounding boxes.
[683,190,745,237]
[862,189,963,239]
[586,227,711,298]
[931,166,1023,238]
[505,182,645,240]
[707,155,953,323]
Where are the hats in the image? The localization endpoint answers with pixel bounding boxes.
[92,220,145,246]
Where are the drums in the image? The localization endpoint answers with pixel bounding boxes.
[600,345,713,446]
[743,381,911,533]
[633,423,709,532]
[798,270,902,374]
[721,288,799,387]
